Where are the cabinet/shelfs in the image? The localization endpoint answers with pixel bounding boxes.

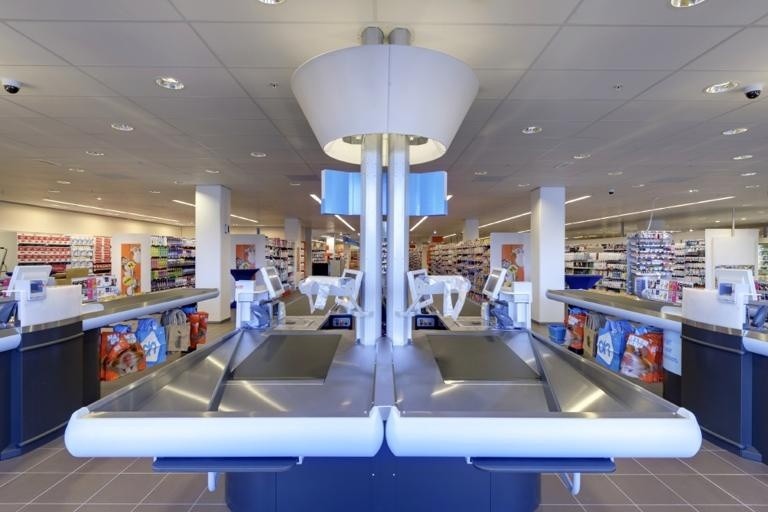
[231,233,329,310]
[564,229,704,305]
[428,237,490,305]
[1,230,198,302]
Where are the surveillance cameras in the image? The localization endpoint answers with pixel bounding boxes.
[608,190,615,195]
[743,85,763,99]
[3,79,22,95]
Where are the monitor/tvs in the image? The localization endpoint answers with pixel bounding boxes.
[716,268,757,303]
[406,268,434,311]
[335,268,363,310]
[255,266,286,301]
[482,267,507,298]
[5,264,53,296]
